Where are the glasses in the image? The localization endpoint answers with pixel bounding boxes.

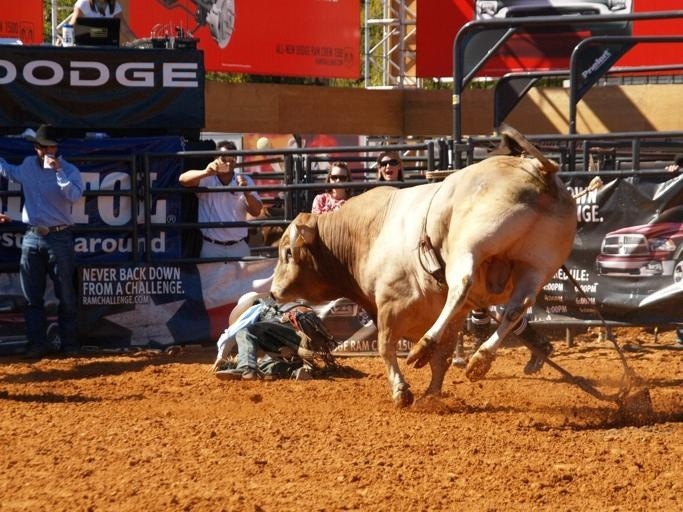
[380,159,398,166]
[331,175,348,182]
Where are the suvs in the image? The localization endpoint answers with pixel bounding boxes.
[595,203,682,283]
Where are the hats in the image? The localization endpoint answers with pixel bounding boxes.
[24,125,71,146]
[229,291,276,326]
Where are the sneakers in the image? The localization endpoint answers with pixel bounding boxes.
[26,345,47,358]
[294,364,312,380]
[523,341,553,374]
[59,345,82,354]
[452,353,476,366]
[216,365,257,381]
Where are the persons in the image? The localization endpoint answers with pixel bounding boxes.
[178,141,263,258]
[69,1,135,40]
[376,152,403,182]
[210,291,330,381]
[0,125,84,358]
[312,162,352,213]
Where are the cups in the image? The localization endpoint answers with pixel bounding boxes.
[63,24,74,47]
[43,153,55,169]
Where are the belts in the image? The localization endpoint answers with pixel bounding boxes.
[203,236,246,244]
[26,224,69,232]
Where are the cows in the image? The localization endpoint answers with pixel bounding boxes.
[269,123,578,408]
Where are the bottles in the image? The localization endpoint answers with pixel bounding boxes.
[85,131,110,138]
